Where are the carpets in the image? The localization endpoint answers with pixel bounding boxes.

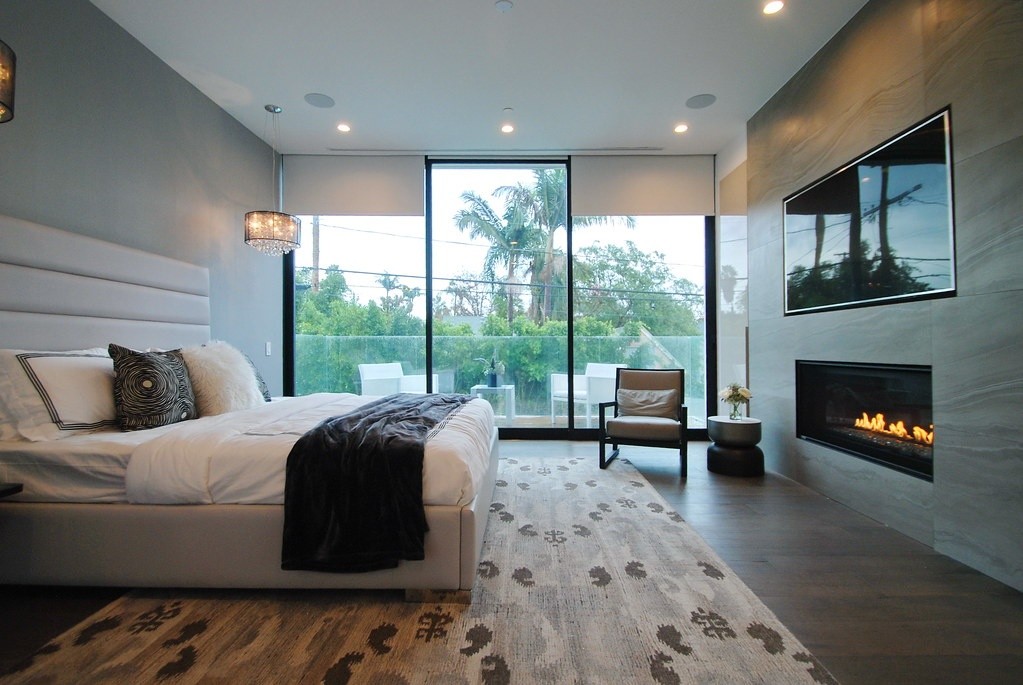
[0,456,840,685]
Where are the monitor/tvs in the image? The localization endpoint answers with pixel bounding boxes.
[783,103,958,317]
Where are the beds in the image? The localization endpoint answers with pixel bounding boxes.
[0,213,501,595]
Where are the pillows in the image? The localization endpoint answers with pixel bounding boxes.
[0,347,113,441]
[109,344,199,430]
[183,340,266,416]
[616,388,678,420]
[241,351,271,402]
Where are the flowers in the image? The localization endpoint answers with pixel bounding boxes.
[719,384,754,417]
[473,350,506,377]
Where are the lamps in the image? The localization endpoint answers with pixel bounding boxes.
[0,40,17,123]
[245,105,301,256]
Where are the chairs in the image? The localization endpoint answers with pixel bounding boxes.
[550,362,628,428]
[357,362,439,395]
[598,367,688,478]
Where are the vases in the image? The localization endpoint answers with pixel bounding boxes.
[487,374,502,387]
[730,407,742,420]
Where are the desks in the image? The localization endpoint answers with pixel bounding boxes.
[705,415,764,477]
[471,385,515,426]
